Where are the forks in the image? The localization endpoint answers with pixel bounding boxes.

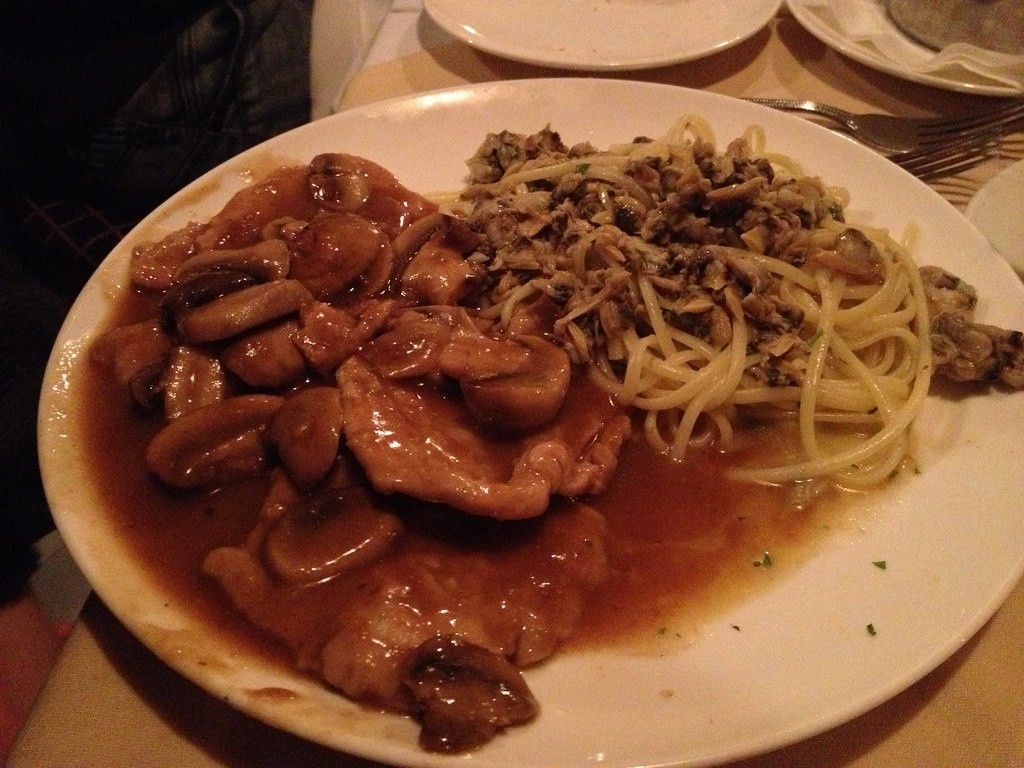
[737,97,1024,155]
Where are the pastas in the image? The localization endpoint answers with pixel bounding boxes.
[430,114,930,489]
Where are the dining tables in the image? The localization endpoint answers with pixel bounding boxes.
[5,2,1024,768]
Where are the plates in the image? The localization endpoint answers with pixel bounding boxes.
[37,78,1024,768]
[965,159,1024,282]
[422,1,783,71]
[785,1,1024,96]
